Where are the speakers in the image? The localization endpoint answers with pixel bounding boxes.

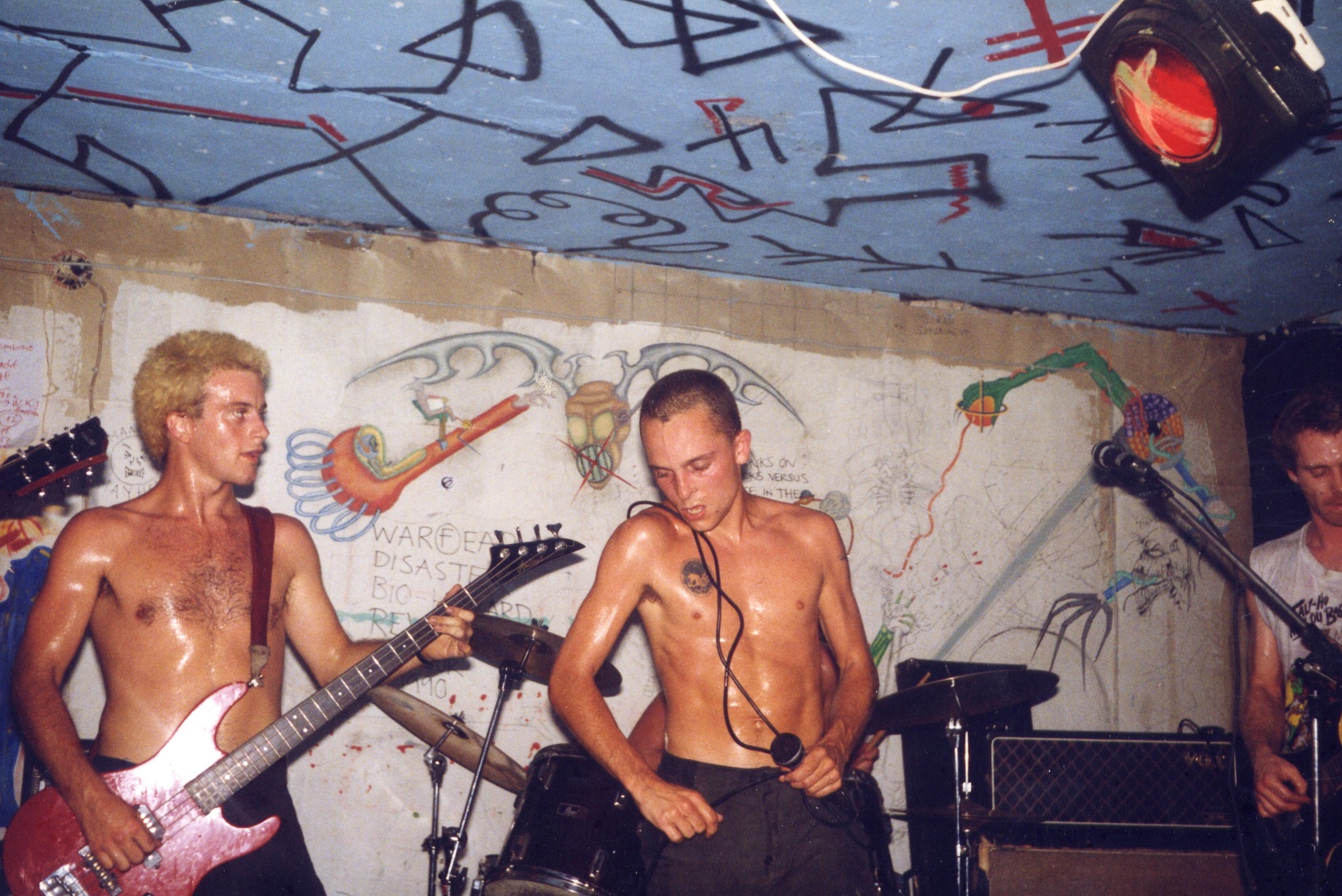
[986,841,1243,896]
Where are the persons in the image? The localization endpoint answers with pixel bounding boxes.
[1241,393,1342,896]
[11,330,478,896]
[549,369,876,896]
[614,627,884,779]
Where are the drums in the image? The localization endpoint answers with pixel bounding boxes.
[481,742,644,896]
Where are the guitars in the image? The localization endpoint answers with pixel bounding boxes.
[1236,760,1342,896]
[0,415,109,522]
[1,522,587,896]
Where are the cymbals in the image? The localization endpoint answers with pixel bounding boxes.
[857,667,1060,736]
[363,685,529,795]
[433,611,623,692]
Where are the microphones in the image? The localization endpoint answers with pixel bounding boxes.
[1091,439,1157,476]
[770,733,858,820]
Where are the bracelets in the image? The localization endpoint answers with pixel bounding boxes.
[416,652,432,665]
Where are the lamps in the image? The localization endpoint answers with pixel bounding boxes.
[1080,0,1330,221]
